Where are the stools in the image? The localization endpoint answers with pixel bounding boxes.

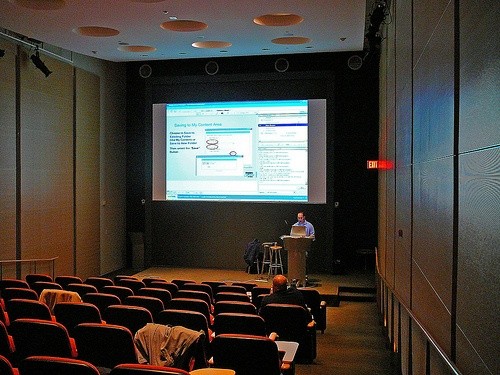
[261,244,283,277]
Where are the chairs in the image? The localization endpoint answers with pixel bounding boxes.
[0,274,326,375]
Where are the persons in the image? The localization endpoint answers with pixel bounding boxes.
[247,318,296,375]
[290,211,315,241]
[258,275,311,319]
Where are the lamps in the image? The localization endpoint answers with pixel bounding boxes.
[30,45,53,78]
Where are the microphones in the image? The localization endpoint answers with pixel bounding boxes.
[285,220,290,233]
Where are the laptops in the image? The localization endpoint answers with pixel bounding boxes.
[292,226,306,237]
